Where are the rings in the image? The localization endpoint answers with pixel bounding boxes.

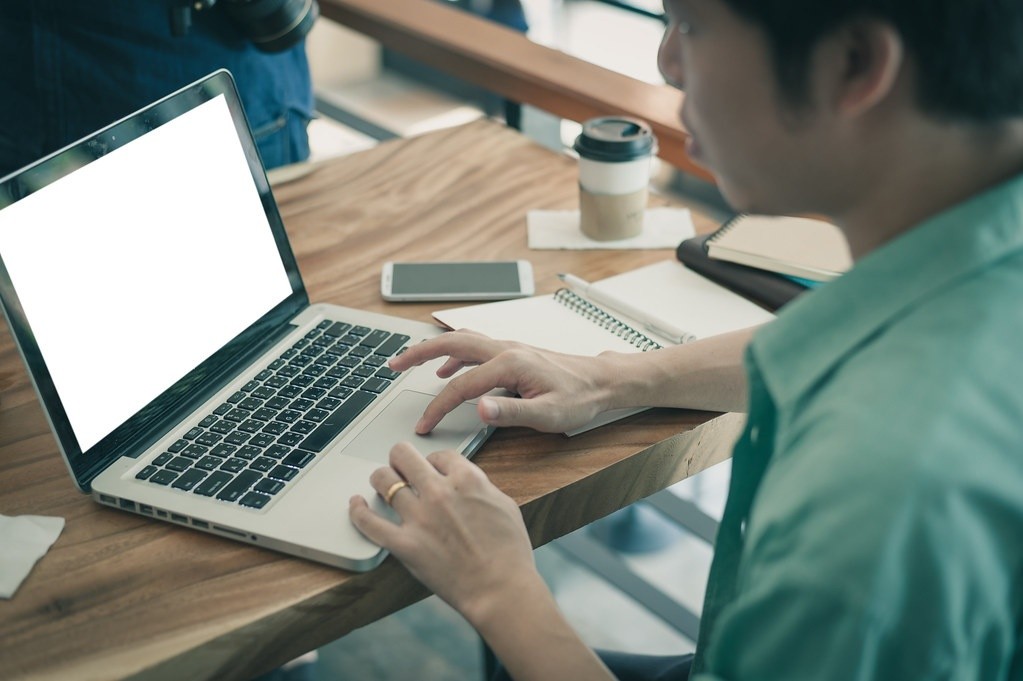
[386,481,411,509]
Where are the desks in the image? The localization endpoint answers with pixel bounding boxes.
[0,113,821,680]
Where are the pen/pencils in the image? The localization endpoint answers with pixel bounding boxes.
[556,272,697,345]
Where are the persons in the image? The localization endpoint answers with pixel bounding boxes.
[350,0,1023,681]
[0,0,529,181]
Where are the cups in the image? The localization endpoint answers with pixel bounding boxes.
[572,115,658,240]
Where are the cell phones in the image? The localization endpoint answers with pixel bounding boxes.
[381,259,536,301]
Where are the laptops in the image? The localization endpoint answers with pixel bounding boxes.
[0,68,516,573]
